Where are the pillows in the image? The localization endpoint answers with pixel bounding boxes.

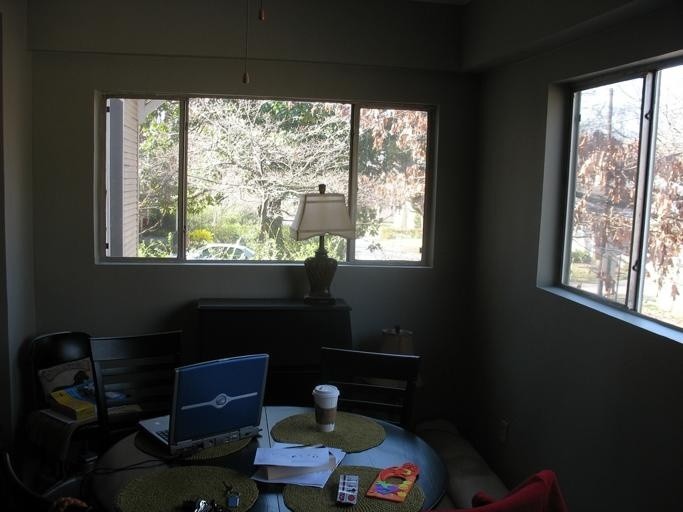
[37,356,95,408]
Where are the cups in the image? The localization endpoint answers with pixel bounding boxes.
[312,384,340,433]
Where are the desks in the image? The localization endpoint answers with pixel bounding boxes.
[92,406,449,512]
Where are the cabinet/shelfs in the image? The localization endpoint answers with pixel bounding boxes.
[198,298,352,408]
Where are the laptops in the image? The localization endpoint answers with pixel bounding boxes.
[138,355,273,454]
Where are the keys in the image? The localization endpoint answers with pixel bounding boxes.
[223,481,235,497]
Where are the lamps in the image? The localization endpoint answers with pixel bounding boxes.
[360,328,426,388]
[296,184,356,305]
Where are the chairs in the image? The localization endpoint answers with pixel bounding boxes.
[88,330,185,455]
[436,470,555,512]
[4,451,108,512]
[321,346,422,433]
[28,331,144,482]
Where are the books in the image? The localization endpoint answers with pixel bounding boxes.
[45,377,126,421]
